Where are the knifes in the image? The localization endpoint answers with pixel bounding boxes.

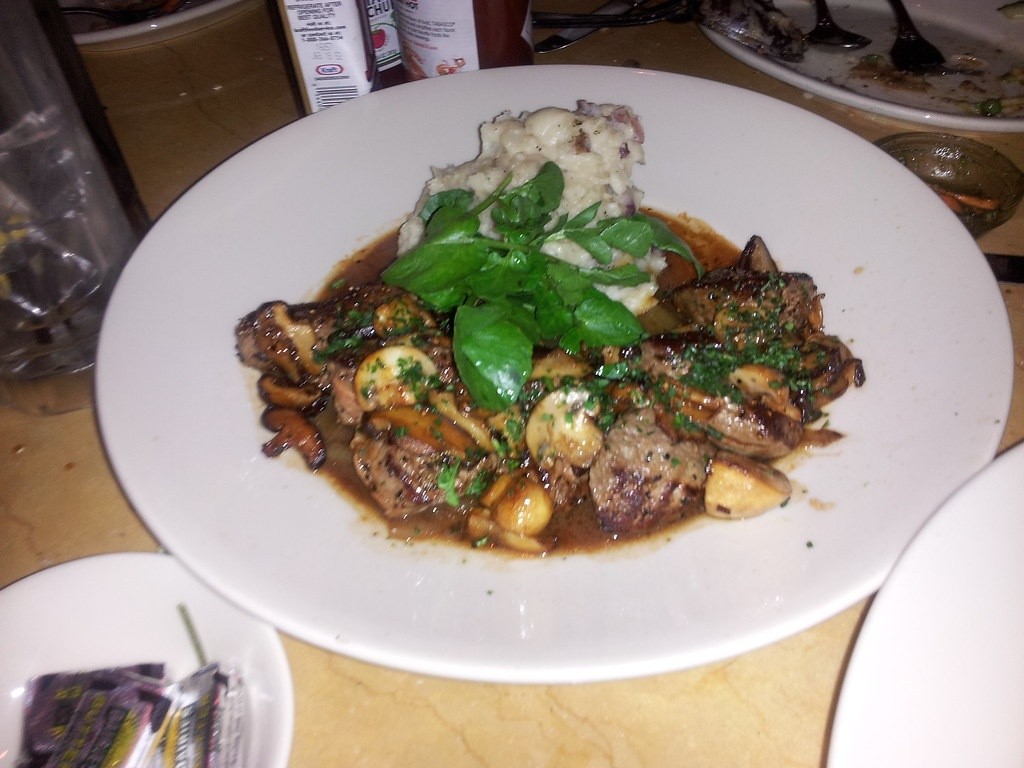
[535,0,649,54]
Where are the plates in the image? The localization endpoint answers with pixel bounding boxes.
[94,63,1013,684]
[699,0,1024,132]
[825,441,1024,768]
[0,551,294,768]
[59,0,265,58]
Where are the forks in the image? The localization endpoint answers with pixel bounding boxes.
[60,0,188,24]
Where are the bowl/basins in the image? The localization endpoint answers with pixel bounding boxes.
[873,131,1024,237]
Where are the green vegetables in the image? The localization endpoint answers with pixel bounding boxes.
[380,163,703,413]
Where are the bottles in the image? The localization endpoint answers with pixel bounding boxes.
[396,0,534,82]
[366,0,406,87]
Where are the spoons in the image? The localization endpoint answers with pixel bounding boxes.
[889,0,951,76]
[801,0,873,50]
[530,0,704,28]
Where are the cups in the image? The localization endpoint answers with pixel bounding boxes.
[0,0,138,417]
